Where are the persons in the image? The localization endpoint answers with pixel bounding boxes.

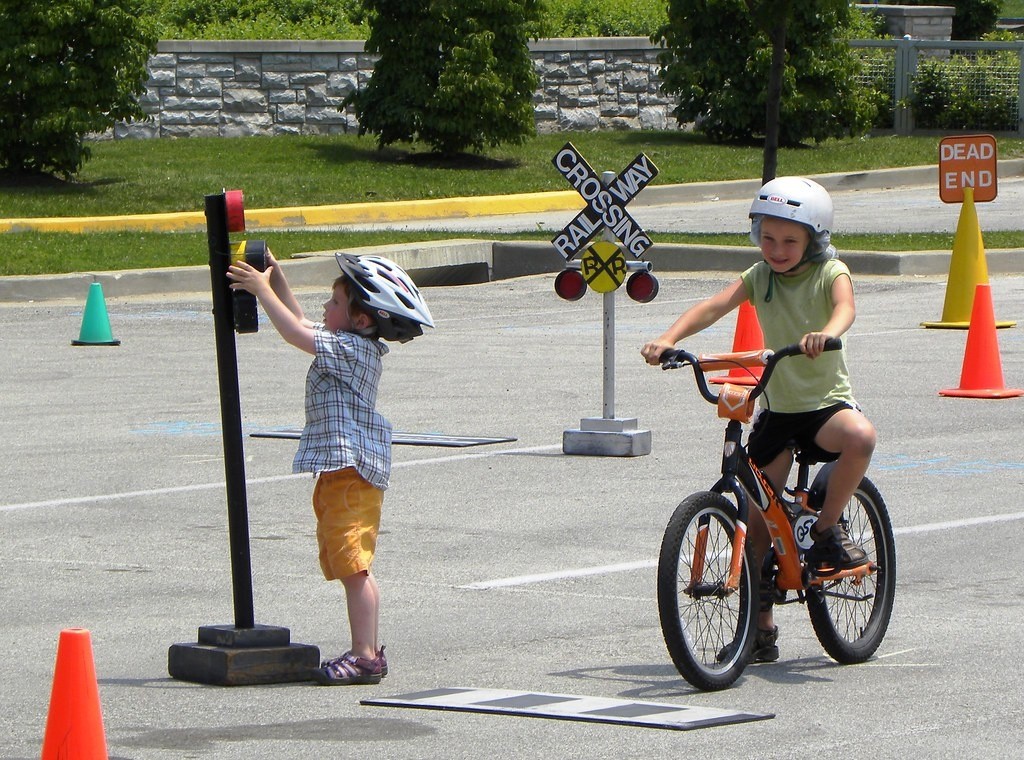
[640,176,882,663]
[228,248,395,685]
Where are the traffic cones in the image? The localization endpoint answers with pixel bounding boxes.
[41,629,110,760]
[707,294,769,384]
[937,284,1024,399]
[918,185,1017,331]
[69,281,122,346]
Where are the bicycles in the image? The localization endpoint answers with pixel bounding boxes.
[643,340,897,692]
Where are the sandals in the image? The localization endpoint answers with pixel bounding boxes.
[804,520,867,569]
[716,626,779,662]
[321,645,388,676]
[311,654,382,685]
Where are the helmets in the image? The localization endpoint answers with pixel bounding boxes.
[748,176,834,236]
[335,252,435,344]
